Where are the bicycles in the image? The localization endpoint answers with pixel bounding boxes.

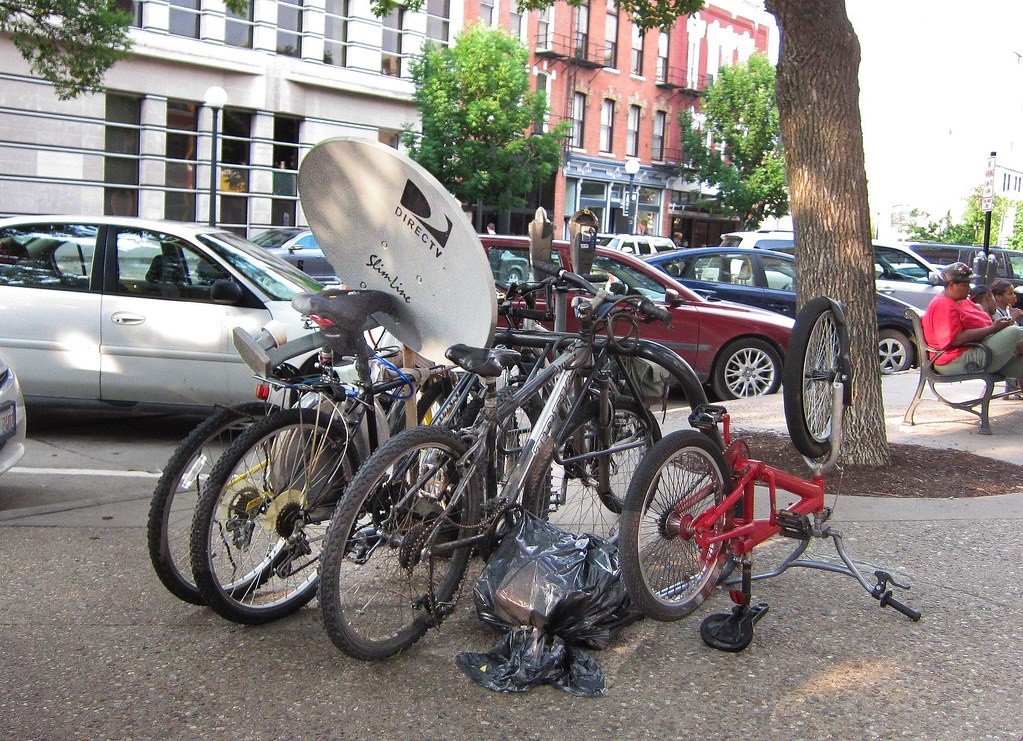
[144,135,922,666]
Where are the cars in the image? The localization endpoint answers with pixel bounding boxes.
[590,229,679,276]
[488,244,531,288]
[620,245,927,374]
[0,212,490,439]
[246,226,345,288]
[696,226,1023,342]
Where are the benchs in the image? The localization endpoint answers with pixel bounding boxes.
[903,308,1004,435]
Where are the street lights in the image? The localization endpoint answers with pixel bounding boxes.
[624,158,641,235]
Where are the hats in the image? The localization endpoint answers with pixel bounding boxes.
[969,285,991,301]
[941,261,981,283]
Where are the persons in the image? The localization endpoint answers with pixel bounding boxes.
[921,261,1023,401]
[673,231,688,250]
[487,223,496,234]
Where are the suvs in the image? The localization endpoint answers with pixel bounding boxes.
[475,228,800,402]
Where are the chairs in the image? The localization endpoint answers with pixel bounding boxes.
[145,254,190,299]
[737,261,753,285]
